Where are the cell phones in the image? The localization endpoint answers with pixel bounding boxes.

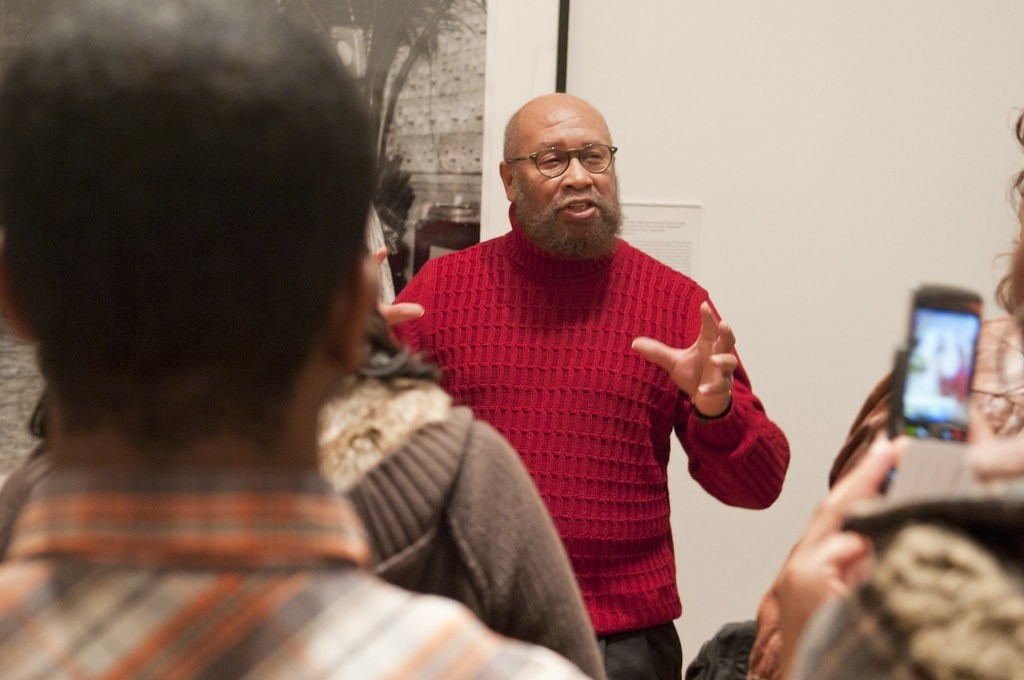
[889,286,983,488]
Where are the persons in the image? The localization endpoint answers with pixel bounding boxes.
[741,110,1024,680]
[0,1,606,680]
[362,92,791,679]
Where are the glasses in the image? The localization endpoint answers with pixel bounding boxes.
[505,144,617,178]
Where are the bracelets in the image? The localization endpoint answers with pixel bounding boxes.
[690,392,733,419]
